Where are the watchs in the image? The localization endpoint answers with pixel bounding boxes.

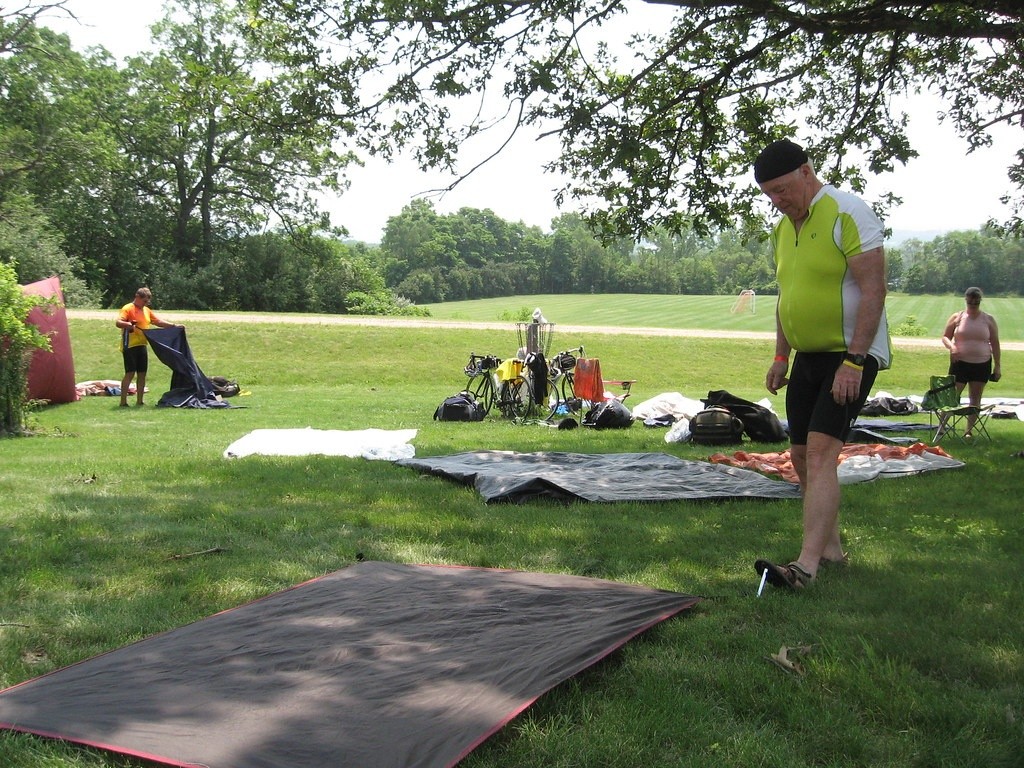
[845,353,866,366]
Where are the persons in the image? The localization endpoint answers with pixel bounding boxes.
[116,287,185,407]
[754,140,894,594]
[935,287,1001,441]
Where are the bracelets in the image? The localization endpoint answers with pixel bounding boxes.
[774,356,789,363]
[843,360,863,371]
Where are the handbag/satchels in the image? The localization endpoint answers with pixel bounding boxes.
[433,390,487,421]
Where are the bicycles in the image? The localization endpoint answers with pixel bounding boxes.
[466,345,595,419]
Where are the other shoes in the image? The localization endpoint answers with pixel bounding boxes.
[136,402,144,404]
[936,432,944,439]
[965,432,972,437]
[120,403,129,406]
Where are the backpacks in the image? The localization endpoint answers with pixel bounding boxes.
[689,405,744,442]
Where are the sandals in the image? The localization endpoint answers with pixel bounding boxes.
[754,558,812,591]
[818,551,848,569]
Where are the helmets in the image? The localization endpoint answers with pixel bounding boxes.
[464,365,482,377]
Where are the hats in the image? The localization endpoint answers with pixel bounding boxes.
[754,140,808,184]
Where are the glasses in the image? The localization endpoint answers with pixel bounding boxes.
[967,300,980,305]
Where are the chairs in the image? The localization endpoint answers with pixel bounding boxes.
[573,357,637,426]
[920,375,996,448]
